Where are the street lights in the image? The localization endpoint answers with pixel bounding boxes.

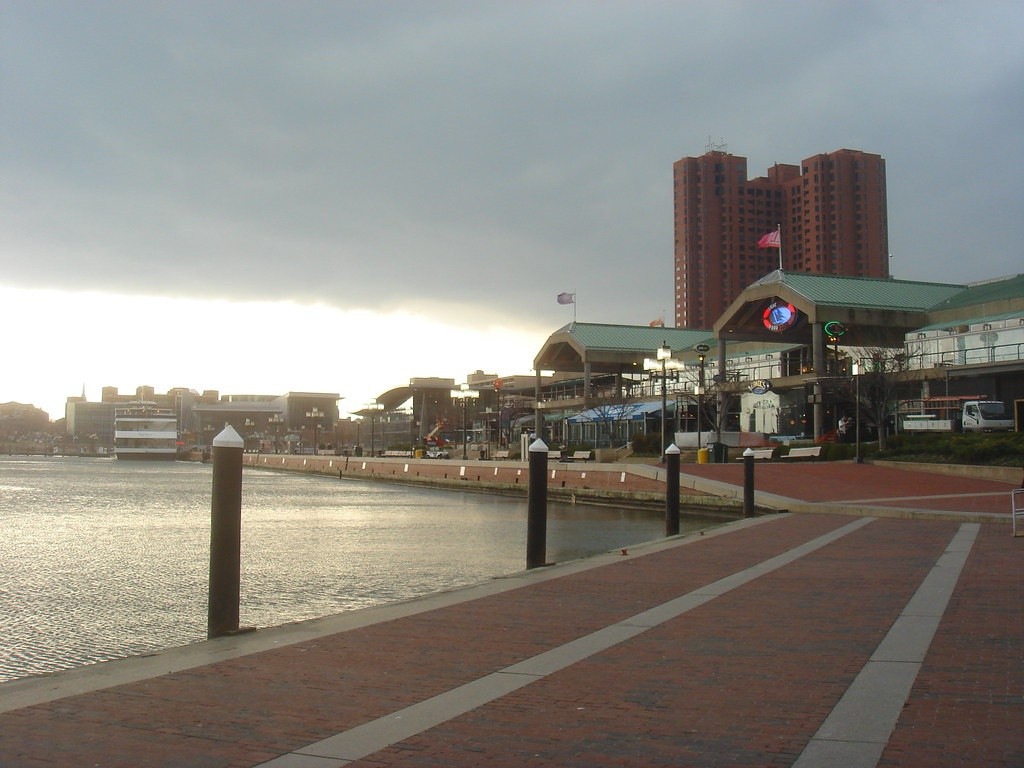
[306,407,323,455]
[657,340,671,464]
[244,418,255,453]
[450,390,479,460]
[695,382,704,450]
[269,413,285,454]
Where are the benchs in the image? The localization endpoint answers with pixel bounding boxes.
[546,450,562,462]
[317,449,336,457]
[380,450,414,459]
[780,446,822,463]
[735,446,774,463]
[567,450,592,463]
[491,450,510,461]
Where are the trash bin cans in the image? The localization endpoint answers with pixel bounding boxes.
[414,445,425,458]
[697,442,729,464]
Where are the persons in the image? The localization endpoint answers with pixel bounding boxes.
[319,443,325,450]
[838,416,849,443]
[327,443,332,450]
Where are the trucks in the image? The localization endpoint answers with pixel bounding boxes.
[903,401,1015,433]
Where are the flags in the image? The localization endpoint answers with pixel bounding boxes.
[757,230,779,248]
[557,293,575,305]
